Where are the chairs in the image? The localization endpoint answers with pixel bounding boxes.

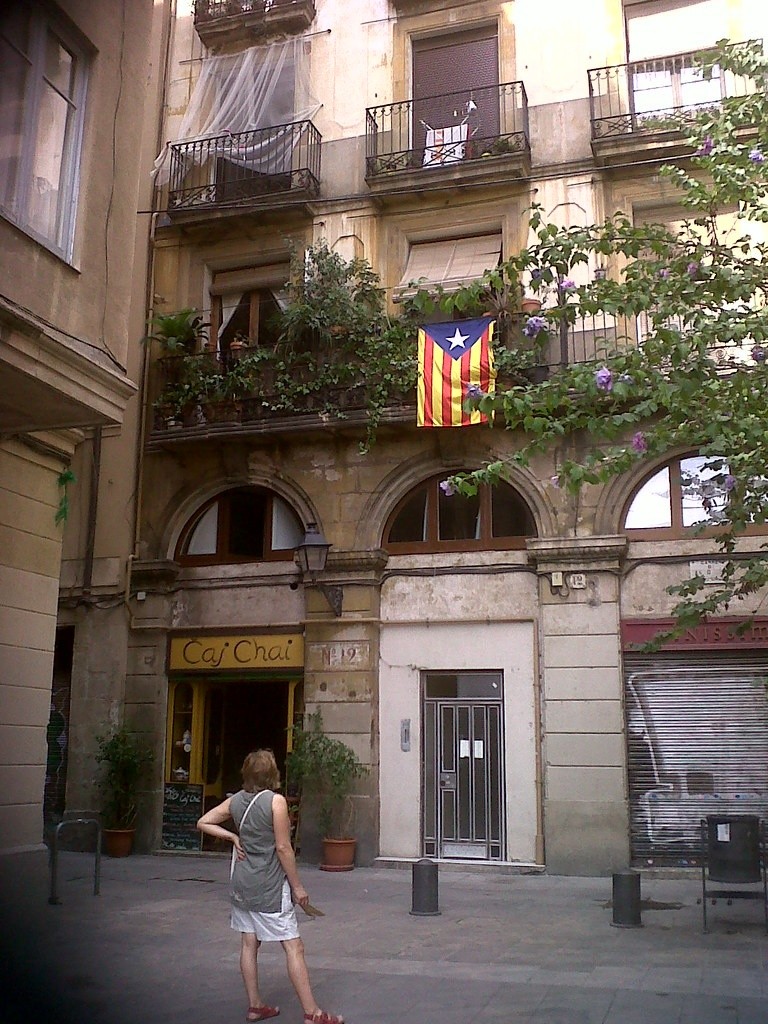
[701,815,768,937]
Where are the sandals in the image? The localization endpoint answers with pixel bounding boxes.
[246,1004,280,1022]
[304,1008,344,1024]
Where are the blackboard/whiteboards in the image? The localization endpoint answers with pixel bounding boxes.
[162,783,205,850]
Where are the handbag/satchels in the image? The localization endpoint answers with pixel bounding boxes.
[230,844,237,878]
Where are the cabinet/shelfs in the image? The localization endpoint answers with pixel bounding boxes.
[172,711,192,781]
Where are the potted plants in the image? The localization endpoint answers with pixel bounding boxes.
[95,717,158,858]
[139,0,551,431]
[281,710,371,865]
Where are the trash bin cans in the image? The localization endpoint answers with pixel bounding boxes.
[700,814,768,933]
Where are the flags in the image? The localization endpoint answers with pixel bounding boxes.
[416,317,497,427]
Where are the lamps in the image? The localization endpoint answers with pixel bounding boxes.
[291,523,343,617]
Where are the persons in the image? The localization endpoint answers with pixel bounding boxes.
[197,751,344,1024]
